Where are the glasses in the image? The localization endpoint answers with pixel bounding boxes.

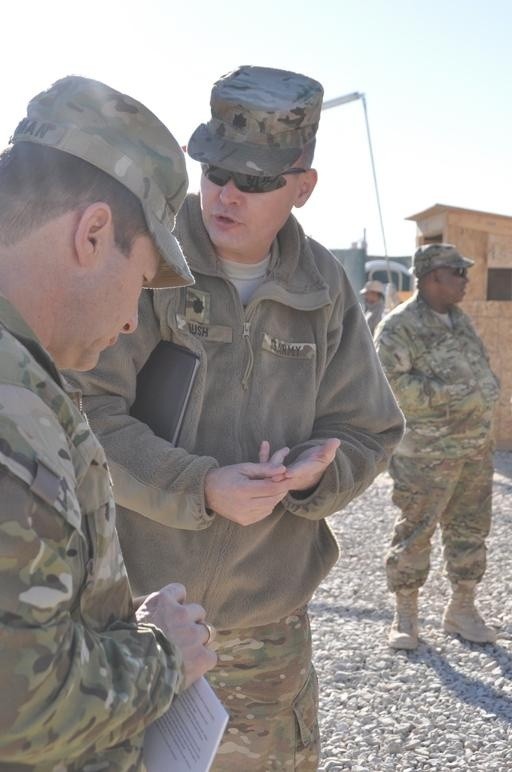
[197,158,307,195]
[448,266,468,279]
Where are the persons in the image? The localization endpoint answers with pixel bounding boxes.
[65,65,412,772]
[1,74,220,772]
[360,280,387,333]
[373,244,504,652]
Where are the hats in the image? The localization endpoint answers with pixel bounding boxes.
[186,65,325,179]
[9,75,196,292]
[359,281,384,295]
[411,242,475,278]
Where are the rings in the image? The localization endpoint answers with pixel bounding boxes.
[198,619,217,647]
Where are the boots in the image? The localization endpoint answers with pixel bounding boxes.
[388,589,419,651]
[442,577,496,644]
